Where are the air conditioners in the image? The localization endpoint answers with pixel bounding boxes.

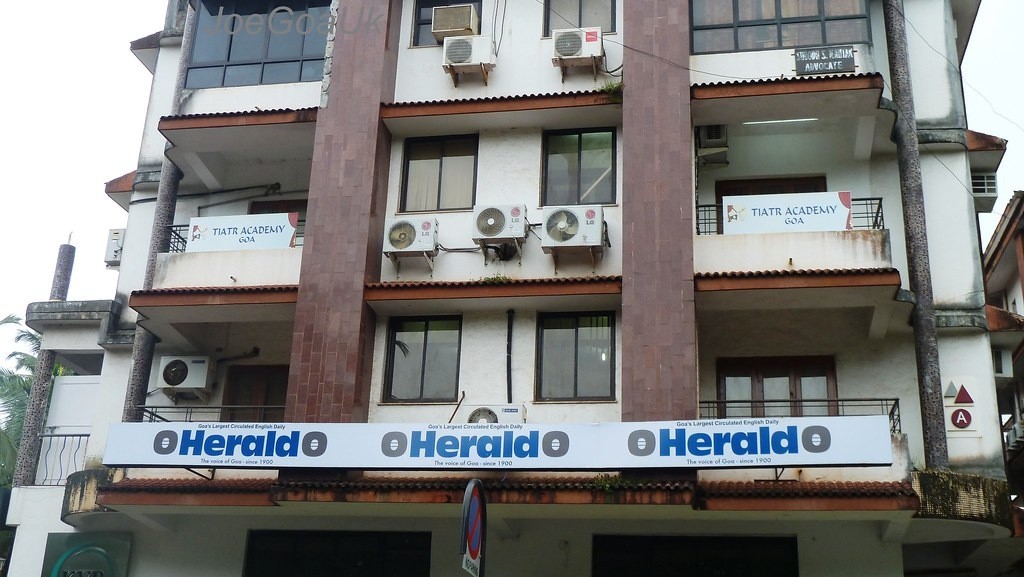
[155,355,218,395]
[471,203,528,243]
[431,4,480,44]
[382,216,440,258]
[442,34,497,73]
[540,205,606,255]
[552,26,604,68]
[461,402,528,425]
